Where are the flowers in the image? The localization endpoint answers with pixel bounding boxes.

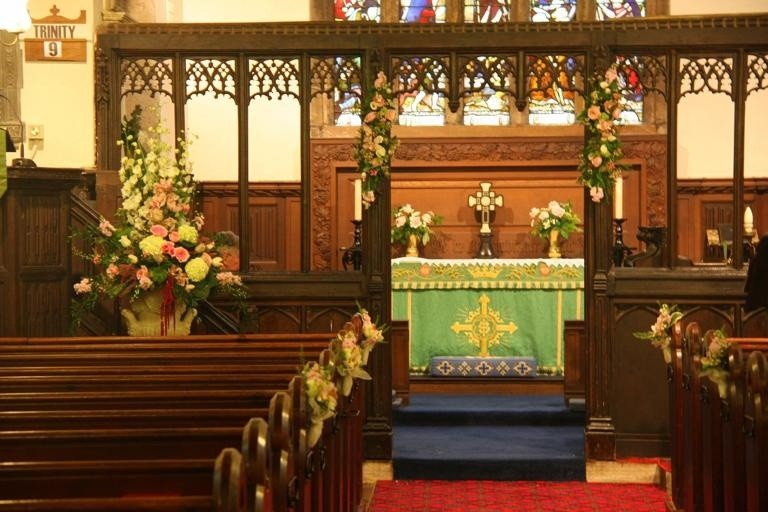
[352,70,403,209]
[575,62,628,205]
[632,300,697,373]
[394,204,445,247]
[528,199,584,243]
[61,102,267,338]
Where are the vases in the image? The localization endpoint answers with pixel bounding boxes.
[407,234,419,256]
[119,292,198,337]
[548,230,562,258]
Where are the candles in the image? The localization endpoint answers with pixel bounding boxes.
[354,179,362,220]
[614,177,623,218]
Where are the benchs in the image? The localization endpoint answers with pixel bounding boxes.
[0,313,364,512]
[665,319,768,512]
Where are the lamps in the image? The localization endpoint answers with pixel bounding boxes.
[0,0,37,168]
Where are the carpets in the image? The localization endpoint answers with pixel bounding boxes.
[363,479,675,512]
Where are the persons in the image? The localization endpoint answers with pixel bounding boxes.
[739,232,768,319]
